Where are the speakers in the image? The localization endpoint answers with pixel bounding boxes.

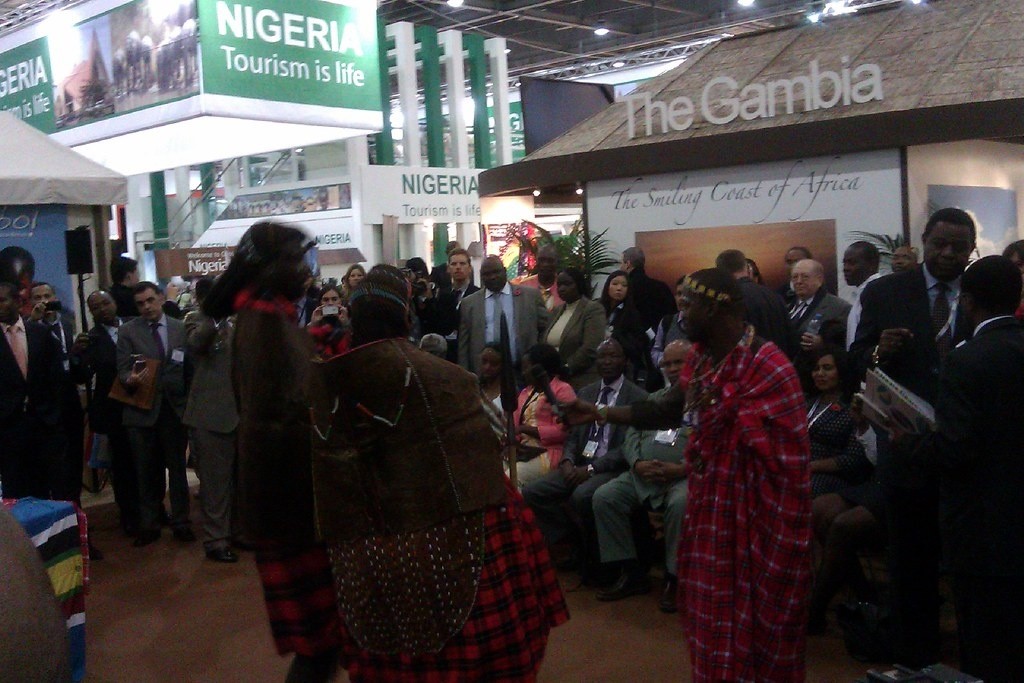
[65,229,93,274]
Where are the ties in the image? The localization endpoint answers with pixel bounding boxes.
[9,326,27,380]
[933,283,951,362]
[594,388,612,458]
[150,323,165,357]
[493,294,504,343]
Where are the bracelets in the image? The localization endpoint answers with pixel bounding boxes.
[597,405,606,419]
[873,345,879,356]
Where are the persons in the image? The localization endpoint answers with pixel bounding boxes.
[311,278,571,683]
[0,247,35,316]
[0,281,103,560]
[556,269,814,683]
[716,208,1023,682]
[202,224,342,683]
[75,258,252,562]
[305,242,697,613]
[26,283,74,376]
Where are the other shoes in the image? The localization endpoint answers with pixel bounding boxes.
[556,554,589,569]
[561,572,603,590]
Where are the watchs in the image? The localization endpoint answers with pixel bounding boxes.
[587,464,595,476]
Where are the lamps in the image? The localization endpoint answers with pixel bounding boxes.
[532,188,542,197]
[575,181,586,194]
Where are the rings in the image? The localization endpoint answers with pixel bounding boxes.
[809,343,812,346]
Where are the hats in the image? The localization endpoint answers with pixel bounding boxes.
[684,269,754,318]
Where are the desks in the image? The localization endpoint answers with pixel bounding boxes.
[0,498,91,683]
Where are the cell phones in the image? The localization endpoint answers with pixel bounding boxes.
[45,301,61,311]
[136,360,146,375]
[322,306,338,316]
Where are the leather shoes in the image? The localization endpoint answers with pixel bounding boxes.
[133,529,161,546]
[661,593,678,613]
[89,536,103,560]
[207,548,238,561]
[597,570,650,601]
[172,527,196,543]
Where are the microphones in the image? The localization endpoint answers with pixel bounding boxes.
[530,364,568,424]
